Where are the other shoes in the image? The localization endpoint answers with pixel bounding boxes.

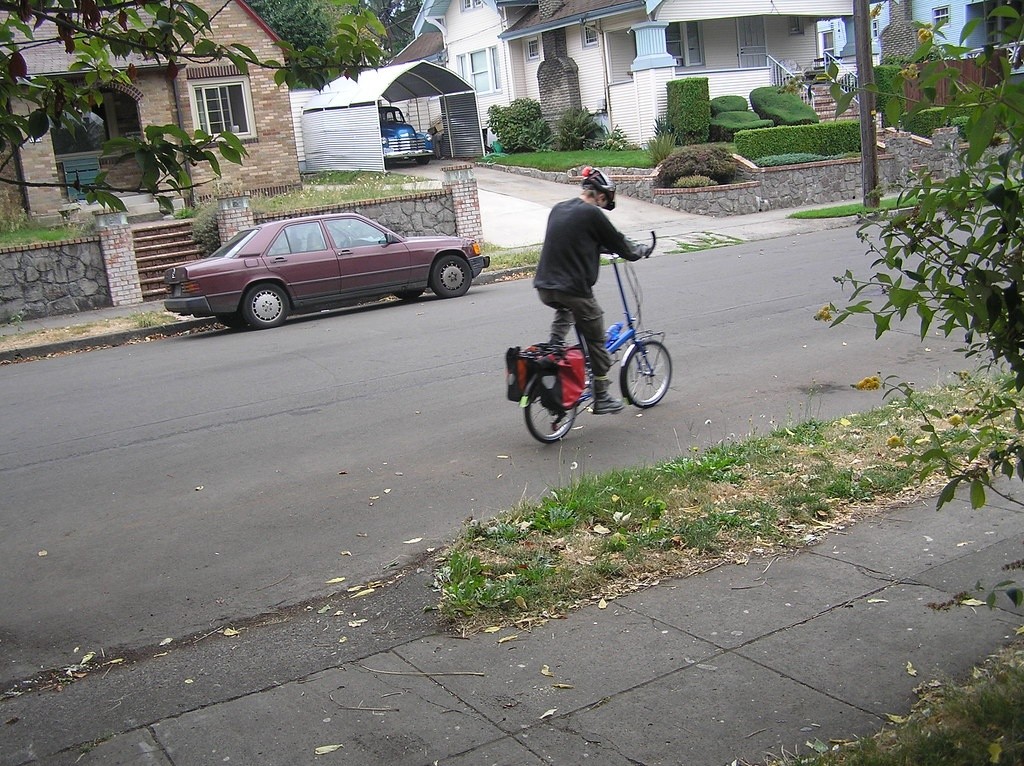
[593,391,623,414]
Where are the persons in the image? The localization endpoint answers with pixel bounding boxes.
[533,167,649,415]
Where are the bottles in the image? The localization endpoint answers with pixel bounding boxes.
[603,322,623,348]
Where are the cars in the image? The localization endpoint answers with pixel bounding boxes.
[378,106,436,170]
[164,213,490,329]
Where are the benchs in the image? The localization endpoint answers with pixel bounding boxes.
[806,57,832,77]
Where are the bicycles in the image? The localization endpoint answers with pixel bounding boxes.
[518,231,672,443]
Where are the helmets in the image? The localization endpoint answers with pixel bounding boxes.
[581,166,617,210]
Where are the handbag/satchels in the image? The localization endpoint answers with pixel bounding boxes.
[506,342,585,411]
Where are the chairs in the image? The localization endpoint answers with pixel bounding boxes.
[290,229,306,252]
[777,58,804,87]
[306,232,323,251]
